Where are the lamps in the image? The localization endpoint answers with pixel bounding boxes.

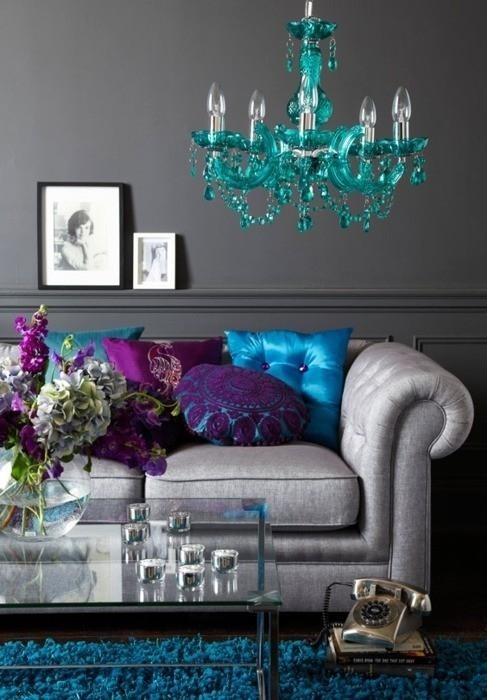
[186,2,429,233]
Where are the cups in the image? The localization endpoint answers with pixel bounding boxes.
[210,548,239,575]
[122,543,147,562]
[167,535,189,549]
[138,584,164,602]
[177,585,204,603]
[121,522,149,547]
[176,564,204,591]
[168,512,193,531]
[127,502,151,524]
[136,559,168,583]
[212,574,239,596]
[178,543,205,564]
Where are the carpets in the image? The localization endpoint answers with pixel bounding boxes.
[1,631,487,699]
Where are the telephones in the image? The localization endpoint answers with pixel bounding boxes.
[340,577,432,652]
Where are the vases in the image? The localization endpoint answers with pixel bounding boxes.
[0,451,92,545]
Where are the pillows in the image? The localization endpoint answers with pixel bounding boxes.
[221,328,353,452]
[172,362,312,452]
[43,325,142,386]
[100,336,222,441]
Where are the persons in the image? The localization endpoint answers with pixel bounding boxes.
[144,244,165,281]
[60,209,94,269]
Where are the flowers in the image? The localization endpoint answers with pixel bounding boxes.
[2,301,185,536]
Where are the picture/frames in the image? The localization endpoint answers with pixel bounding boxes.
[35,179,131,292]
[129,229,180,291]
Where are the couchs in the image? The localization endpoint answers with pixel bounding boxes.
[3,331,475,619]
